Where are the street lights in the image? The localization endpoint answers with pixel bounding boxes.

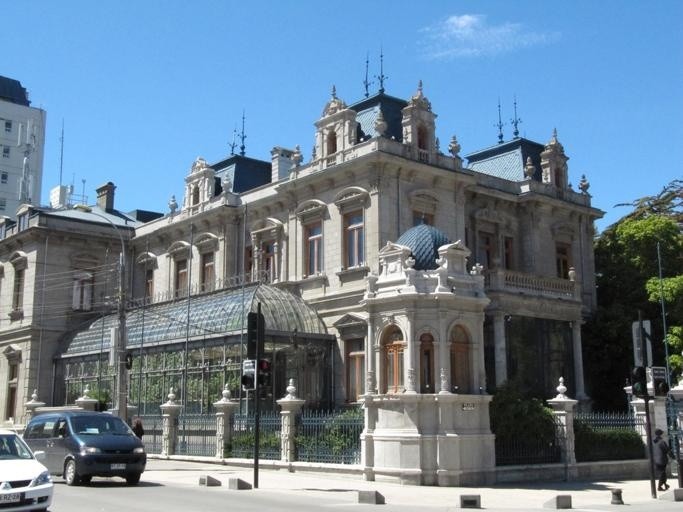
[72,203,126,423]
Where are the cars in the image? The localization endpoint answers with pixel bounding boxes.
[0,427,54,512]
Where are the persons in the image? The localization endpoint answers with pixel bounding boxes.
[653,428,676,492]
[132,418,144,440]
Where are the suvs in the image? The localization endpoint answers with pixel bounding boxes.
[22,409,147,487]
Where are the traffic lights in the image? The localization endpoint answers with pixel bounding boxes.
[240,371,255,390]
[258,358,273,388]
[632,366,647,395]
[654,377,670,396]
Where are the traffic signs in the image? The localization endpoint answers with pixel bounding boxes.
[664,400,682,436]
